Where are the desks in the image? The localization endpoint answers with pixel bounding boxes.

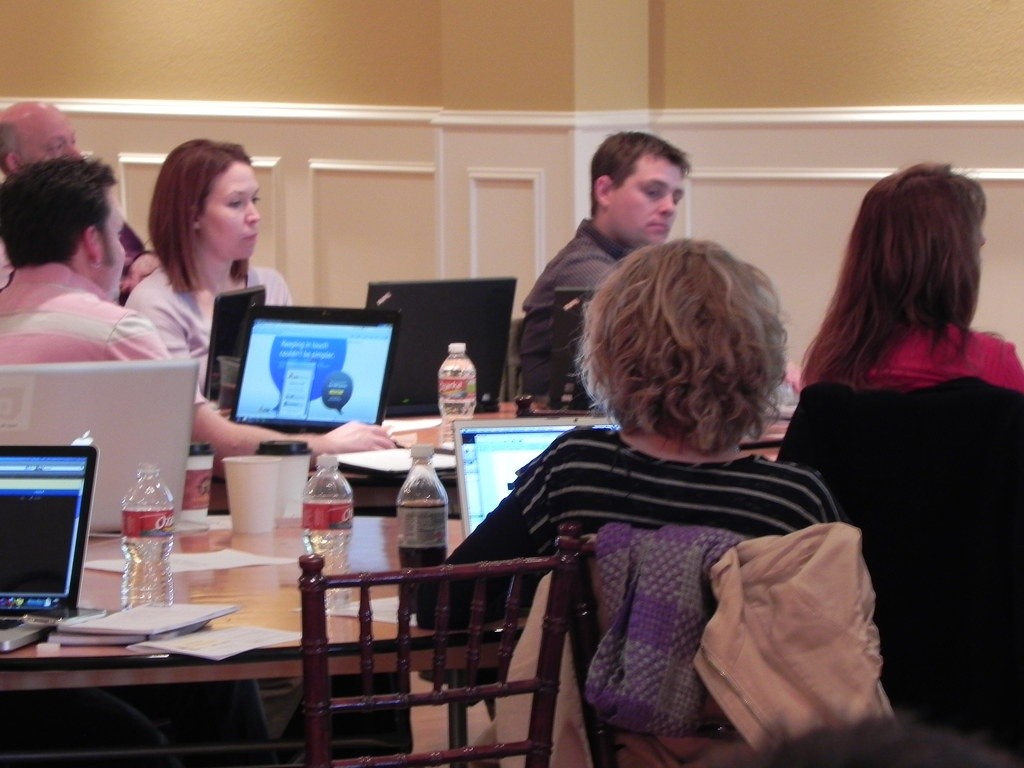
[0,394,802,768]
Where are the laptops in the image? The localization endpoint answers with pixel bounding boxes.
[456,415,626,543]
[363,276,519,418]
[0,359,201,651]
[228,303,405,439]
[190,285,268,457]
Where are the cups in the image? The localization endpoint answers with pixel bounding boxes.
[253,439,312,521]
[177,440,217,523]
[221,455,283,535]
[217,355,241,417]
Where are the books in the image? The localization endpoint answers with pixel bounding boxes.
[48,602,240,644]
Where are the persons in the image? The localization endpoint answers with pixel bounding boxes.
[126,147,399,479]
[517,131,692,410]
[414,234,850,768]
[697,704,1018,768]
[0,147,173,366]
[774,164,1024,750]
[0,101,159,295]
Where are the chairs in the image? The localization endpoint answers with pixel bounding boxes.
[558,521,862,768]
[298,536,582,768]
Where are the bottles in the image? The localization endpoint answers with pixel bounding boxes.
[119,463,176,611]
[437,342,477,450]
[301,455,353,611]
[395,443,448,630]
